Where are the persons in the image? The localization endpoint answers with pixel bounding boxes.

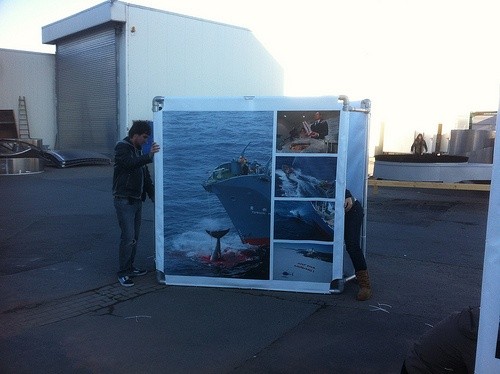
[411,134,428,155]
[294,112,328,152]
[113,120,160,287]
[345,190,374,300]
[239,156,263,174]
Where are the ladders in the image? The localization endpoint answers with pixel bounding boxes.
[18,95,30,138]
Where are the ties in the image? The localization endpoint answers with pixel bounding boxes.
[316,121,318,126]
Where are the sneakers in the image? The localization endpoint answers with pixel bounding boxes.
[117,275,135,287]
[128,267,147,276]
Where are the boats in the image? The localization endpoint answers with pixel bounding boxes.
[198,141,271,246]
[309,201,334,240]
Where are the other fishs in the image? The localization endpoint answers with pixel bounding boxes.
[205,228,229,262]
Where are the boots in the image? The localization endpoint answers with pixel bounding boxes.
[355,270,374,301]
